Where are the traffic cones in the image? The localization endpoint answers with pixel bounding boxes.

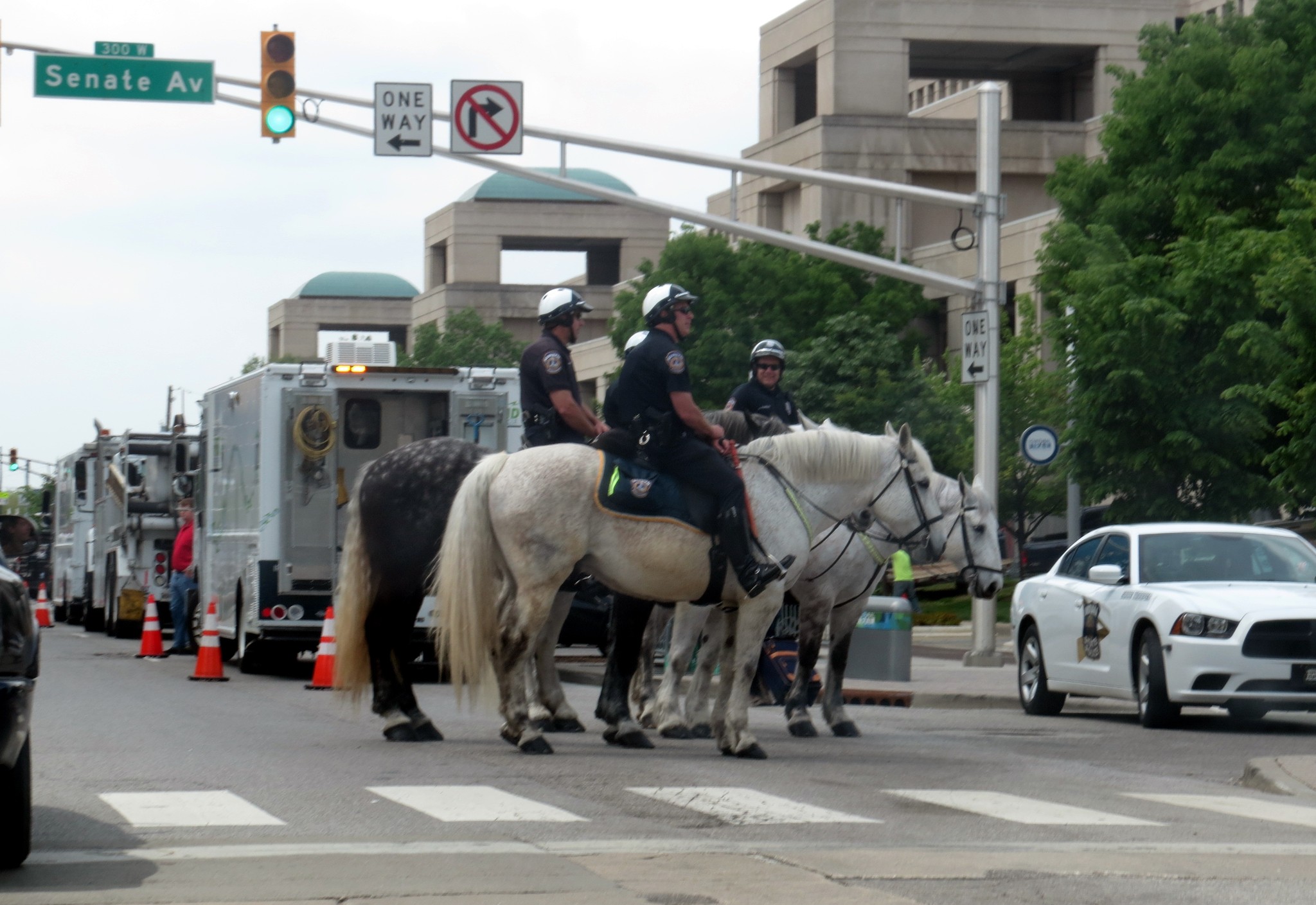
[35,582,57,628]
[304,605,336,691]
[132,594,169,658]
[188,601,231,682]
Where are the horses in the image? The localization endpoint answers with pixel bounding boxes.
[423,417,947,759]
[329,407,1004,741]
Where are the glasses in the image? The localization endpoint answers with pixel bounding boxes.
[758,363,782,370]
[666,306,690,313]
[572,312,580,320]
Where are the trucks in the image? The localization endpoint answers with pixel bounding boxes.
[41,386,201,642]
[170,336,529,674]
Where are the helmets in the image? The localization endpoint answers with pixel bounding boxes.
[623,330,649,354]
[642,283,698,324]
[750,339,786,375]
[538,288,593,322]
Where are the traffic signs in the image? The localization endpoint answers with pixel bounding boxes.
[960,309,991,385]
[375,81,433,156]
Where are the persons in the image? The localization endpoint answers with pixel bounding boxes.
[613,283,798,599]
[725,338,798,425]
[517,286,611,450]
[603,331,650,427]
[163,497,199,655]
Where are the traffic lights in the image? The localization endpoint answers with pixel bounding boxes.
[9,448,20,472]
[261,31,297,139]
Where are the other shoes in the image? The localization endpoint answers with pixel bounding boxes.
[164,647,185,654]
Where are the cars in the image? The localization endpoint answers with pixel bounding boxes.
[0,513,43,873]
[1009,520,1316,731]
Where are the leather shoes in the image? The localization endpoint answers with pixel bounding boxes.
[748,553,796,599]
